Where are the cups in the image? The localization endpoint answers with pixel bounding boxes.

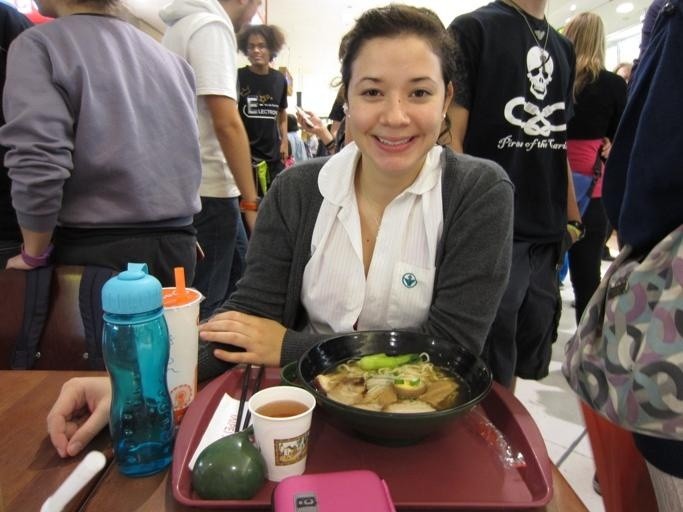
[246,386,316,483]
[161,287,202,433]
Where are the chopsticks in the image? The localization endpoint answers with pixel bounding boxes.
[235,363,265,432]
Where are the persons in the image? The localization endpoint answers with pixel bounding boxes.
[47,3,513,458]
[440,2,682,496]
[0,3,344,321]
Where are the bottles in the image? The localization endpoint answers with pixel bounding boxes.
[99,261,175,478]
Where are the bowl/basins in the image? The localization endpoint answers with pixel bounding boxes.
[280,360,300,387]
[295,329,493,448]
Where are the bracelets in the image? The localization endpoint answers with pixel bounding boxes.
[21,243,54,267]
[327,143,336,150]
[325,139,335,147]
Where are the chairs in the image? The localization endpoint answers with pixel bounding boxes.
[1,263,130,370]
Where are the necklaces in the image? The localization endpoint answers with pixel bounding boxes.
[511,4,549,72]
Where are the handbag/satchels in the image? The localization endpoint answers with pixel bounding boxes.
[558,171,596,281]
[561,224,683,441]
